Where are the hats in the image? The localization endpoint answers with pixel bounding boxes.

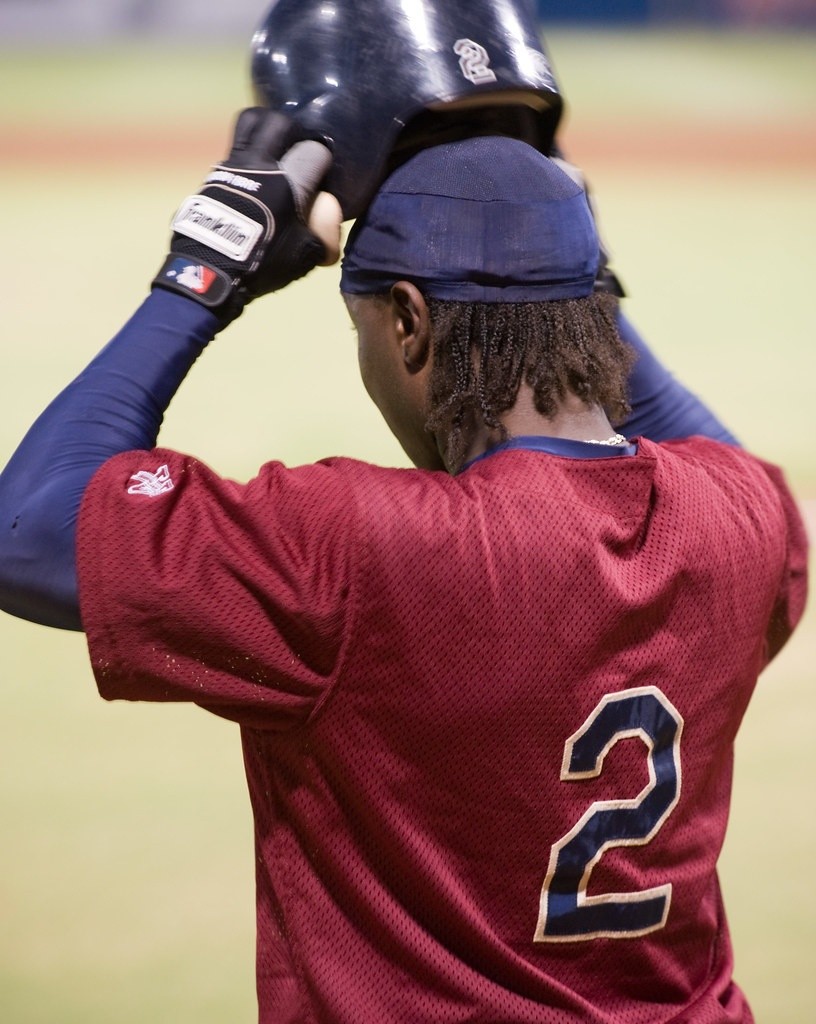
[339,137,598,301]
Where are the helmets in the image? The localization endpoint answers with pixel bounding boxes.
[251,0,562,221]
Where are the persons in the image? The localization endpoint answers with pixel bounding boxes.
[0,109,808,1024]
